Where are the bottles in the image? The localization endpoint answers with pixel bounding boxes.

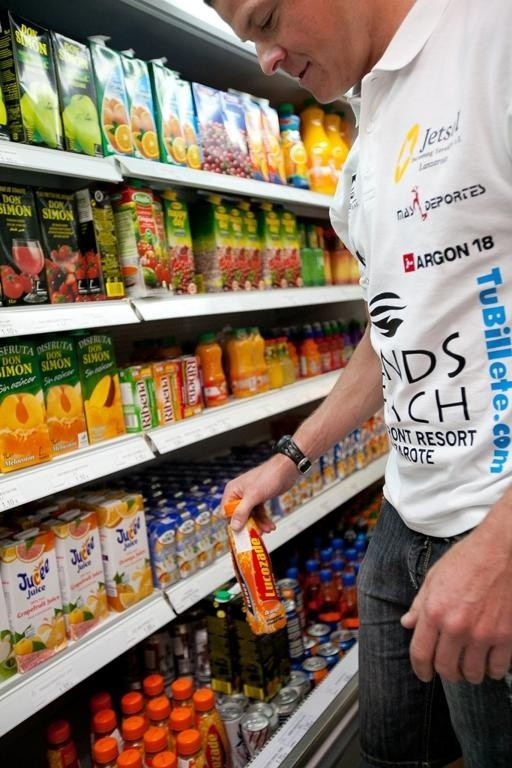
[351,246,362,284]
[288,535,369,619]
[371,497,378,511]
[334,236,352,282]
[369,512,378,527]
[300,105,332,194]
[297,223,314,289]
[324,111,350,193]
[309,225,326,288]
[299,315,361,378]
[226,329,256,395]
[224,504,287,634]
[318,227,332,284]
[262,323,298,387]
[361,504,368,518]
[280,103,309,189]
[241,329,270,391]
[198,336,230,408]
[45,675,234,763]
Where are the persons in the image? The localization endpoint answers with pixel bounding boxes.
[202,0,512,768]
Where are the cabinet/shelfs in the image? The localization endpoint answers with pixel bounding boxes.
[0,0,390,768]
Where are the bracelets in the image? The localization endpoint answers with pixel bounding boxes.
[271,434,312,475]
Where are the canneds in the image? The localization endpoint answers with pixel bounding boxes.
[124,579,356,766]
